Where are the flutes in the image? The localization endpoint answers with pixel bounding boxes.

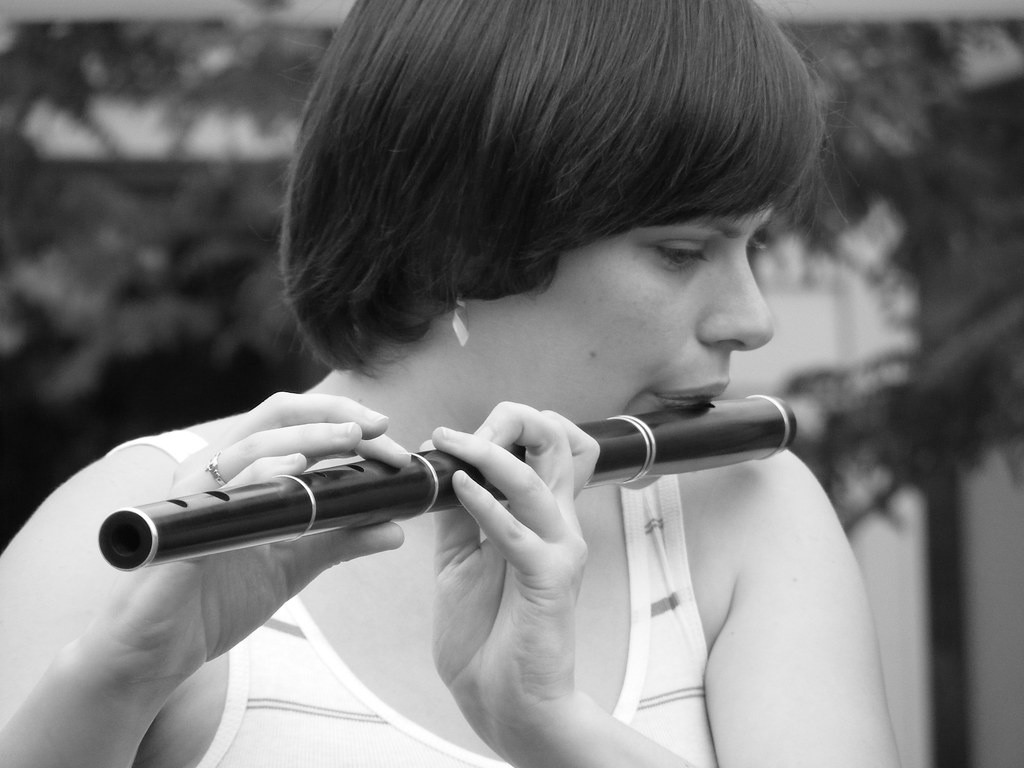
[98,392,799,572]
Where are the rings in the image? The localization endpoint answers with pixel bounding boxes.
[205,451,226,486]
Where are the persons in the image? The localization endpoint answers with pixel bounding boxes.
[0,0,908,768]
